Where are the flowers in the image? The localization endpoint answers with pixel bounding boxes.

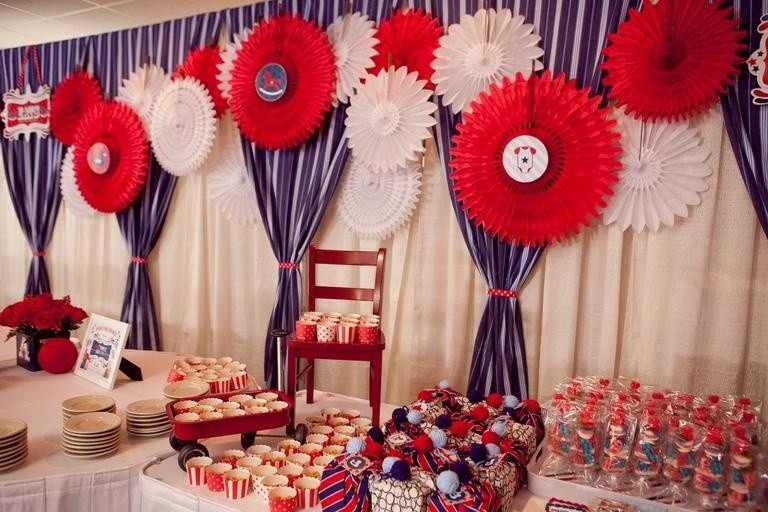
[0,292,88,342]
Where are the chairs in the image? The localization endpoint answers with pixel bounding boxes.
[286,244,386,437]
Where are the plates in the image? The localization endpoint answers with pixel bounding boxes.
[61,379,211,459]
[0,417,29,472]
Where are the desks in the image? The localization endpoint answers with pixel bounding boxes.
[140,389,549,511]
[1,350,187,512]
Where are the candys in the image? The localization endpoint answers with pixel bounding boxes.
[547,374,768,512]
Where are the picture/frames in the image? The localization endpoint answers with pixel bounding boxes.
[73,314,144,389]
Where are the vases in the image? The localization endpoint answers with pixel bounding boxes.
[17,331,71,372]
